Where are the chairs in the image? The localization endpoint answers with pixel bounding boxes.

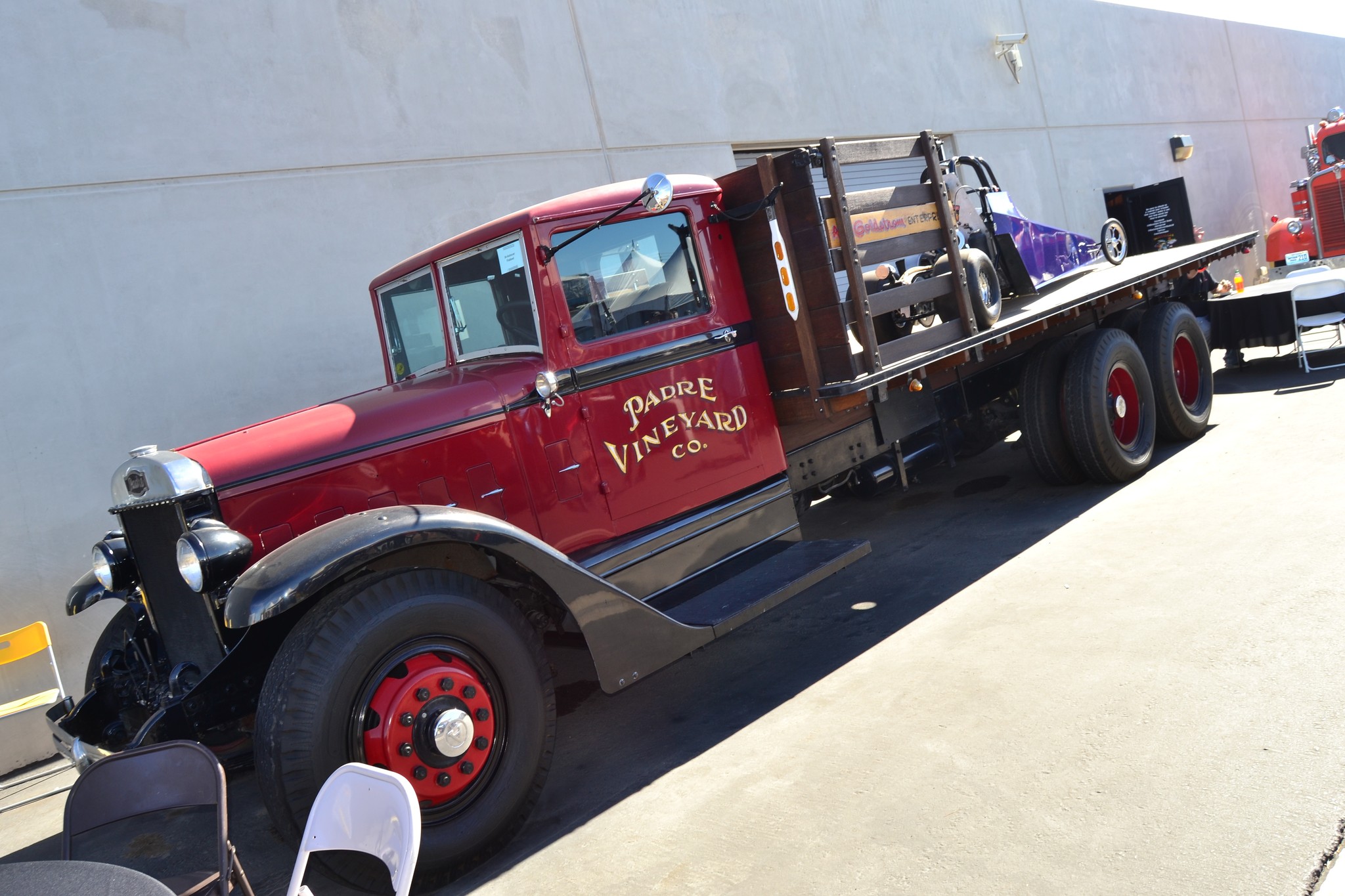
[58,737,258,895]
[284,760,426,894]
[2,619,88,816]
[1289,279,1345,373]
[1285,267,1343,353]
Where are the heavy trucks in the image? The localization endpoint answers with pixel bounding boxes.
[41,123,1257,881]
[1264,107,1345,266]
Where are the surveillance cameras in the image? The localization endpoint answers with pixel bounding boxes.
[995,29,1028,45]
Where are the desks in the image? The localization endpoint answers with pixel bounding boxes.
[1206,265,1345,371]
[3,861,180,896]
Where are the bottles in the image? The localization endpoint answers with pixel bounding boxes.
[1234,270,1243,293]
